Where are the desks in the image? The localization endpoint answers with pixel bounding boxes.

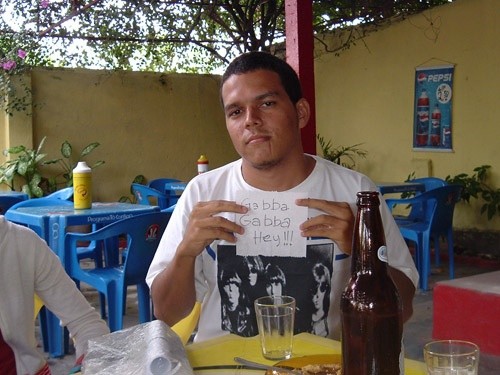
[371,181,426,197]
[181,332,430,375]
[5,201,161,357]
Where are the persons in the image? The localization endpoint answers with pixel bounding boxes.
[144,52,420,343]
[0,216,110,375]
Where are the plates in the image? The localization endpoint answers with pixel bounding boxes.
[266,354,341,375]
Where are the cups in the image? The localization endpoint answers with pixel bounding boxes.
[254,295,296,360]
[423,340,480,375]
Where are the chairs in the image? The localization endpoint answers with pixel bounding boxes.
[0,178,188,357]
[384,178,465,291]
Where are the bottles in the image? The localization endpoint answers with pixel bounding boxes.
[442,124,451,147]
[430,104,441,147]
[339,192,404,375]
[417,89,429,146]
[73,161,92,209]
[198,155,208,174]
